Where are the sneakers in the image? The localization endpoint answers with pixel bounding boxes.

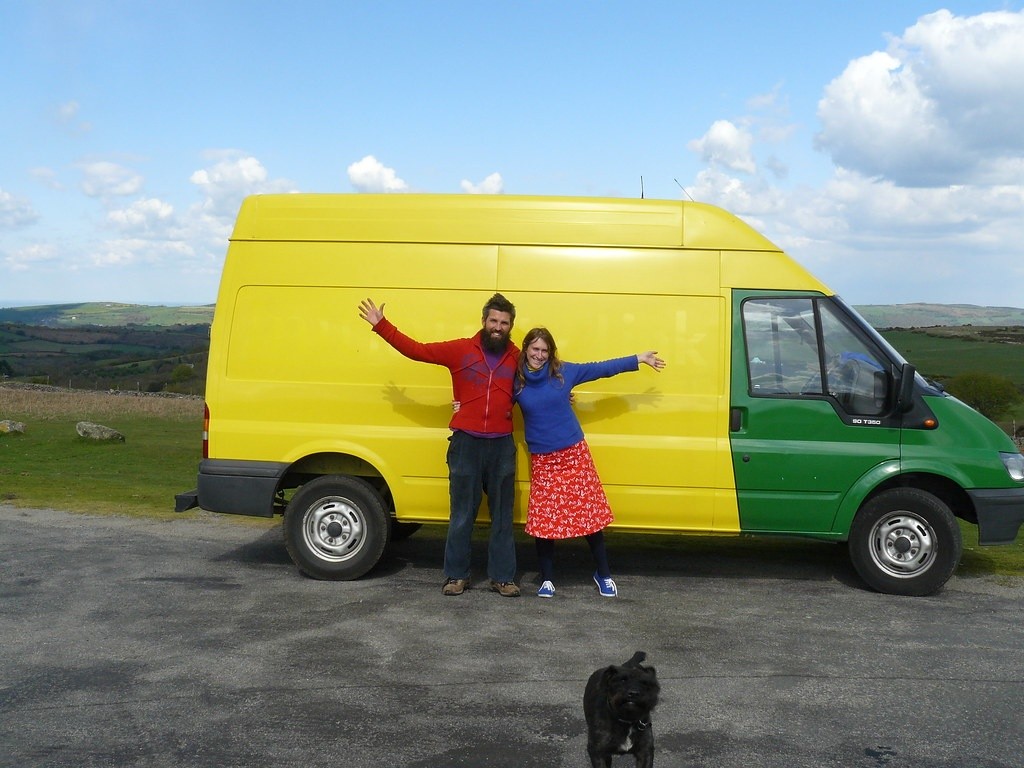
[538,580,555,598]
[593,569,618,597]
[490,578,521,597]
[441,571,473,594]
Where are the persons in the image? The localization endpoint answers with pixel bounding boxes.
[358,293,575,597]
[452,328,666,597]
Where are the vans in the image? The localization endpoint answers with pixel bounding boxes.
[175,191,1024,597]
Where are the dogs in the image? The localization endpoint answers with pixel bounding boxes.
[583,650,662,768]
